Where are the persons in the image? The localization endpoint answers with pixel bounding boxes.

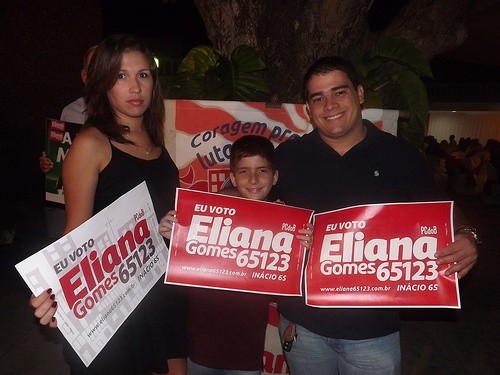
[29,33,188,375]
[429,133,497,183]
[277,55,479,375]
[40,46,97,172]
[160,135,314,375]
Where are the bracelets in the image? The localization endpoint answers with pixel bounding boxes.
[461,226,485,246]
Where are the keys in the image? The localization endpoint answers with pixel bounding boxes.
[283,324,296,351]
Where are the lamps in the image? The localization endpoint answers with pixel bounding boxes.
[264,93,282,108]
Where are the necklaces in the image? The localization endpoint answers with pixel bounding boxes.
[125,134,153,155]
